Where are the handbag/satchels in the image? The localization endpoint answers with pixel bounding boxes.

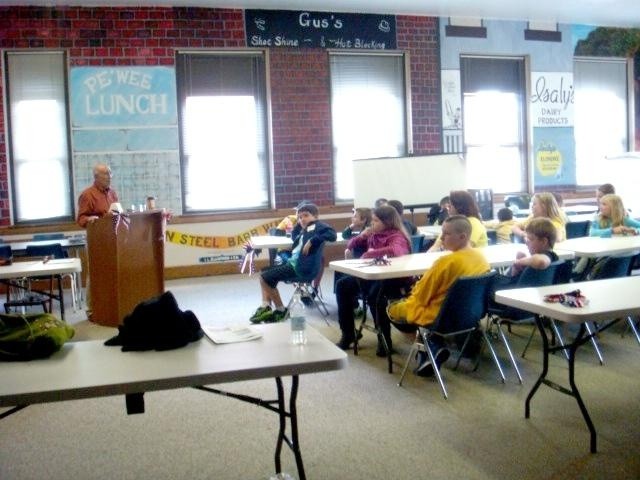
[0,310,75,362]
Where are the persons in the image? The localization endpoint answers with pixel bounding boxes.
[250,203,336,323]
[376,199,388,208]
[428,196,450,226]
[512,192,567,243]
[388,200,417,237]
[596,183,614,211]
[337,205,411,359]
[490,218,558,306]
[486,208,525,243]
[278,201,324,305]
[343,206,373,238]
[575,194,640,278]
[427,191,488,253]
[77,165,119,321]
[386,216,491,377]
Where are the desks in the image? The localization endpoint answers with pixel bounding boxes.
[253,226,435,325]
[2,319,346,478]
[0,234,89,323]
[493,275,640,454]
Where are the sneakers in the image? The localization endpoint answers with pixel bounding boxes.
[416,348,450,377]
[249,306,273,323]
[376,333,392,358]
[336,330,363,350]
[300,297,313,306]
[264,308,289,323]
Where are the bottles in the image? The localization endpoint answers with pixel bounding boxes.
[290,294,305,343]
[475,190,493,212]
[147,197,156,212]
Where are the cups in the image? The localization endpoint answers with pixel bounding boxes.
[140,204,146,212]
[131,205,138,212]
